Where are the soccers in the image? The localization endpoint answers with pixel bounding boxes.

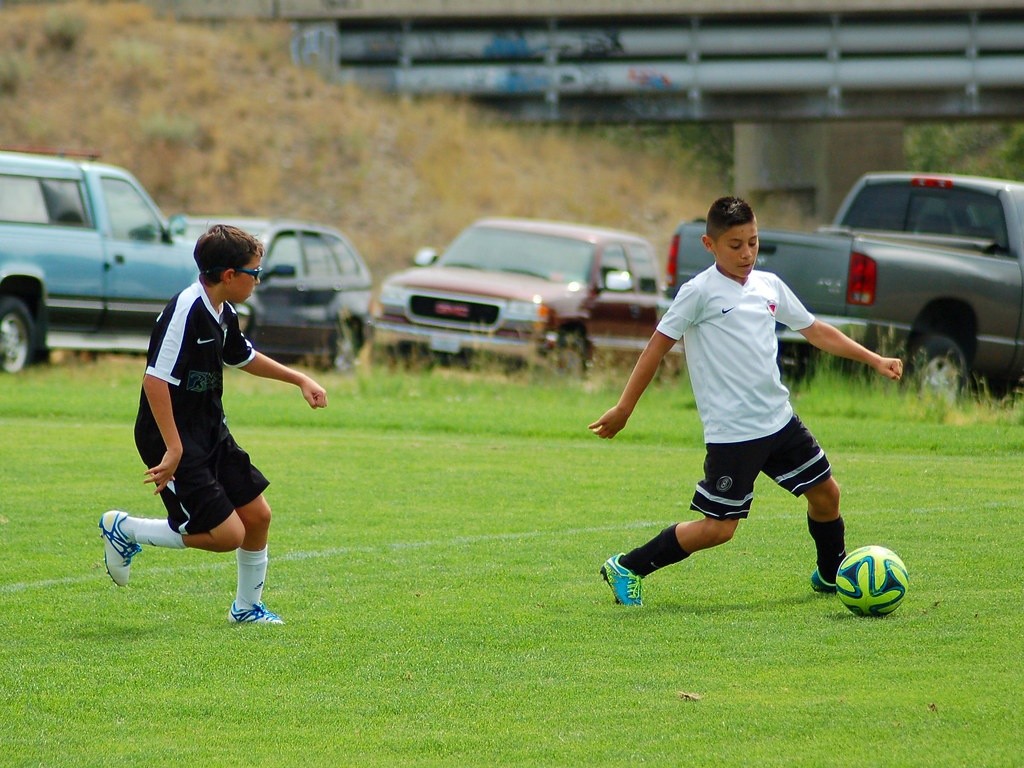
[836,544,911,617]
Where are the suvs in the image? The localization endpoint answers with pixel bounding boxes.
[363,215,663,378]
[175,215,374,369]
[0,147,254,375]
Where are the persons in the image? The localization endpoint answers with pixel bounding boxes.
[589,197,903,606]
[97,224,329,626]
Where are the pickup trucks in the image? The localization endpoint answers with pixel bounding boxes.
[659,170,1024,406]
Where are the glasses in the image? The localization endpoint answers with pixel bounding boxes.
[235,266,261,280]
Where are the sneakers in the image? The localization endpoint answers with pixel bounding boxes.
[599,553,644,606]
[810,570,836,592]
[99,510,142,587]
[228,600,286,624]
